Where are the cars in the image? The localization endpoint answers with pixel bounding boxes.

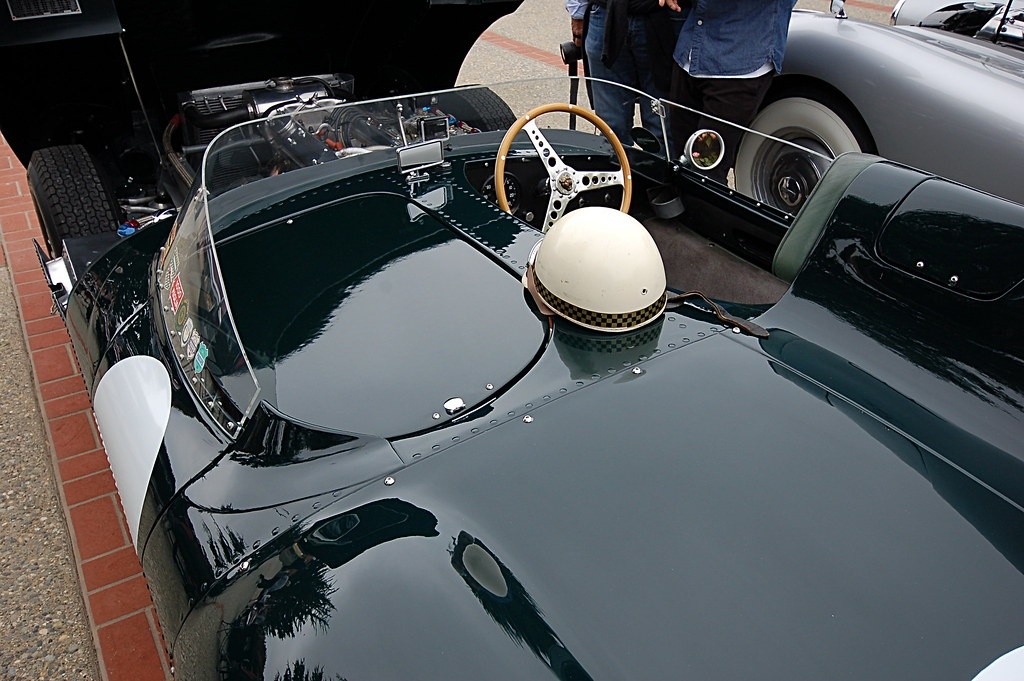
[731,1,1022,215]
[0,0,1024,681]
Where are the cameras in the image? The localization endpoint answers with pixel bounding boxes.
[560,39,585,64]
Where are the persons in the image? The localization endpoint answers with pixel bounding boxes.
[566,0,673,156]
[660,0,799,185]
